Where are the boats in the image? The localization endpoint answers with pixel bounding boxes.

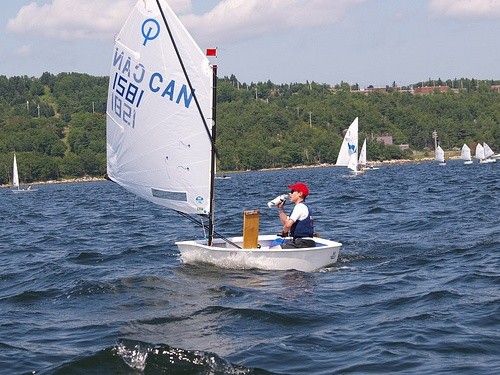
[215,176,232,180]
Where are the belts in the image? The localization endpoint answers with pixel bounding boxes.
[302,237,315,242]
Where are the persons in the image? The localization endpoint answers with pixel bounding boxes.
[269,182,316,249]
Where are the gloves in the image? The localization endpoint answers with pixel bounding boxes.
[281,231,288,237]
[278,199,286,208]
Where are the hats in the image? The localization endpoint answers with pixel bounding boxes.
[288,183,308,197]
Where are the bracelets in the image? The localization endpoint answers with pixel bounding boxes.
[278,209,284,213]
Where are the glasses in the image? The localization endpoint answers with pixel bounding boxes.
[291,190,298,194]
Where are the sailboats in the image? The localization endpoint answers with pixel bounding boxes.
[334,116,379,175]
[12,151,39,193]
[104,0,342,271]
[435,144,448,165]
[461,142,474,165]
[475,141,497,163]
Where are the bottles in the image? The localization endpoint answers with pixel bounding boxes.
[266,192,291,208]
